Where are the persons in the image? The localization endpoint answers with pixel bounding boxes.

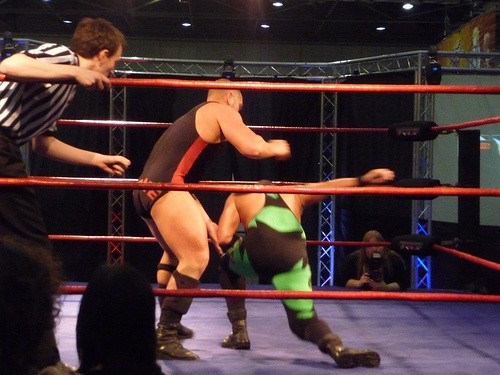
[133,78,292,360]
[71,264,164,375]
[0,234,76,375]
[217,163,395,368]
[343,229,411,290]
[0,18,125,375]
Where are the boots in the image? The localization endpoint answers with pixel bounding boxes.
[157,326,199,361]
[318,333,380,368]
[177,322,193,338]
[222,309,250,350]
